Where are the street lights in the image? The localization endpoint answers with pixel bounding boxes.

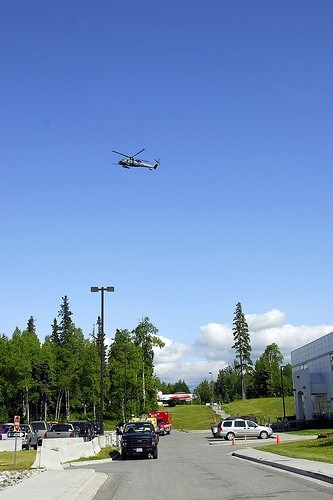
[208,371,214,407]
[280,365,286,417]
[90,286,115,434]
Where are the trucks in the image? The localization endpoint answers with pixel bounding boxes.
[148,410,173,436]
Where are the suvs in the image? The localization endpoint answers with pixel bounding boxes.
[215,418,273,441]
[117,421,163,460]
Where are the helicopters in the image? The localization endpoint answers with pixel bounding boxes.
[111,148,161,171]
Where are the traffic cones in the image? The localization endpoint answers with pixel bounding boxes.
[231,437,237,446]
[275,434,281,445]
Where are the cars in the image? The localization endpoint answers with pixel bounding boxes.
[0,421,105,451]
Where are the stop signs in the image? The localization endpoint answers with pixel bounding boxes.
[14,416,20,431]
